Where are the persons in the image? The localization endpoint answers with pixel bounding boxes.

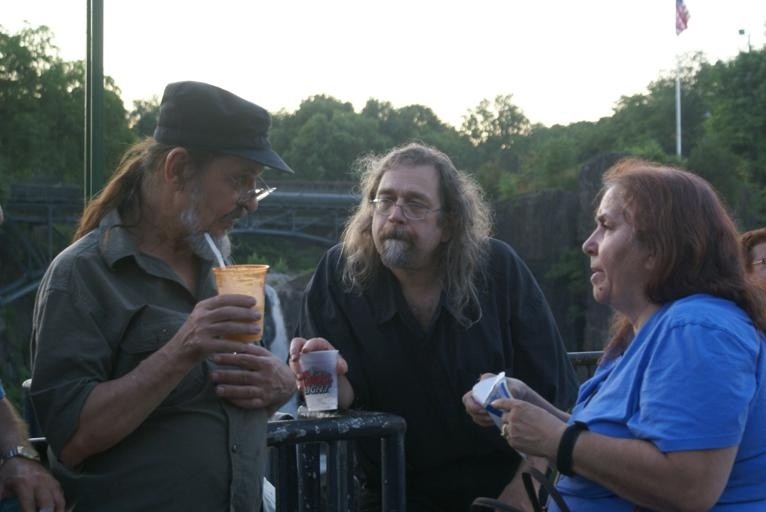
[736,228,766,335]
[0,385,65,512]
[30,81,298,512]
[290,142,580,510]
[464,160,766,512]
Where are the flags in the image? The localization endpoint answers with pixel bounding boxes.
[675,0,689,35]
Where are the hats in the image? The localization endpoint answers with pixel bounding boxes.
[153,81,295,174]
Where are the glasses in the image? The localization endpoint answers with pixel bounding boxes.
[213,154,277,206]
[750,258,766,266]
[369,199,444,220]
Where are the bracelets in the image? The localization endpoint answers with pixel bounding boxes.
[556,420,590,479]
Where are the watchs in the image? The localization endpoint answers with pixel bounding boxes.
[0,446,41,462]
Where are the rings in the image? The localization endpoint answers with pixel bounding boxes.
[500,422,507,436]
